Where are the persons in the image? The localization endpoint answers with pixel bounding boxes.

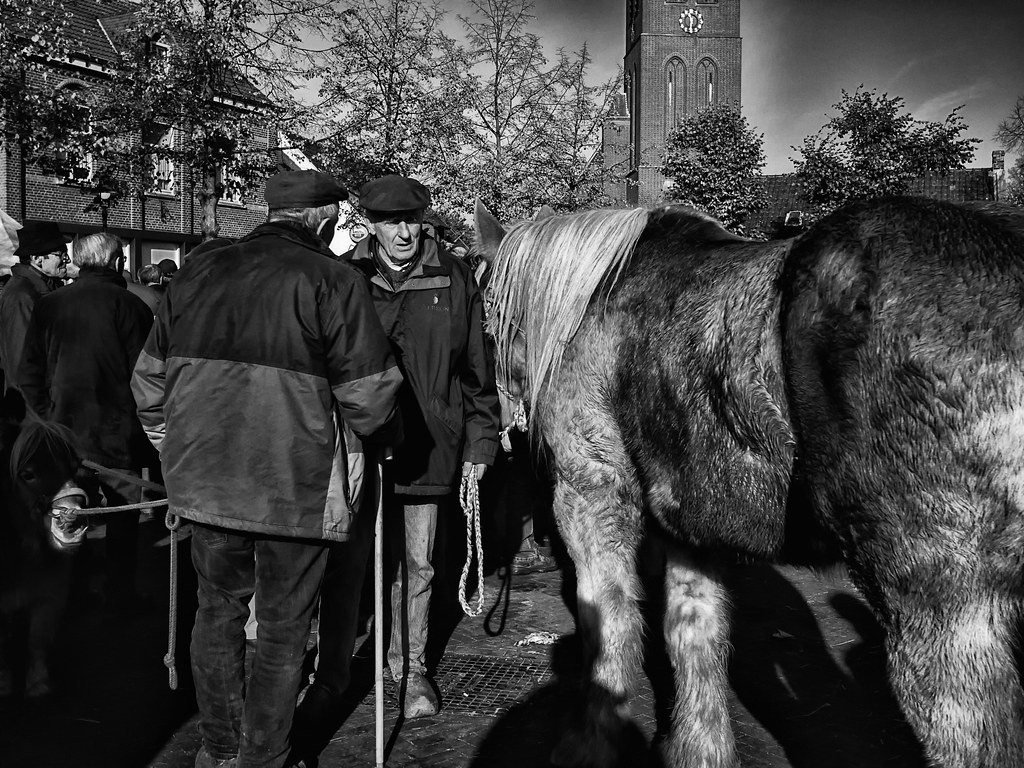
[1,169,562,768]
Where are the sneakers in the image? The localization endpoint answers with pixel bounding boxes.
[509,539,561,576]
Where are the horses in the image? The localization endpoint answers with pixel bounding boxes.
[473,195,1024,768]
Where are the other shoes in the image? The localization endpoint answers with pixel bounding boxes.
[314,663,350,705]
[195,743,306,768]
[396,674,439,718]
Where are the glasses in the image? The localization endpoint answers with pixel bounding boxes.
[112,256,126,263]
[50,252,72,263]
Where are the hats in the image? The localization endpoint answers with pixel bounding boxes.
[264,170,349,209]
[13,222,67,256]
[358,174,430,211]
